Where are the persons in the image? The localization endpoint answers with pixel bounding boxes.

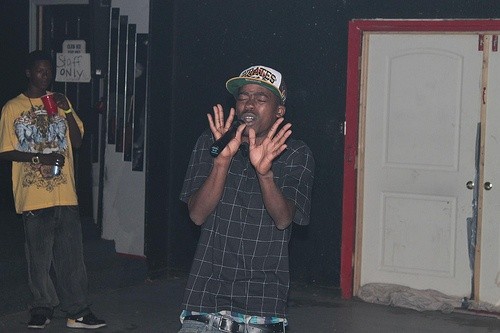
[177,64,315,333]
[1,49,108,330]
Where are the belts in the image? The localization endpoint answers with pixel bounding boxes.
[184,313,285,332]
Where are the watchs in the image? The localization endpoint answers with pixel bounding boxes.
[32,152,41,166]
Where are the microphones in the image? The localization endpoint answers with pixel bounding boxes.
[210,120,245,157]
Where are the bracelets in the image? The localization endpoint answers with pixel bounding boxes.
[64,107,73,116]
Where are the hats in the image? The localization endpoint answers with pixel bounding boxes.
[225,65,288,105]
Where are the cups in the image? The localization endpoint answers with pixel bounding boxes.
[41,93,58,116]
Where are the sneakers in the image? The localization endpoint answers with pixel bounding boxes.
[67,311,108,329]
[27,313,50,329]
